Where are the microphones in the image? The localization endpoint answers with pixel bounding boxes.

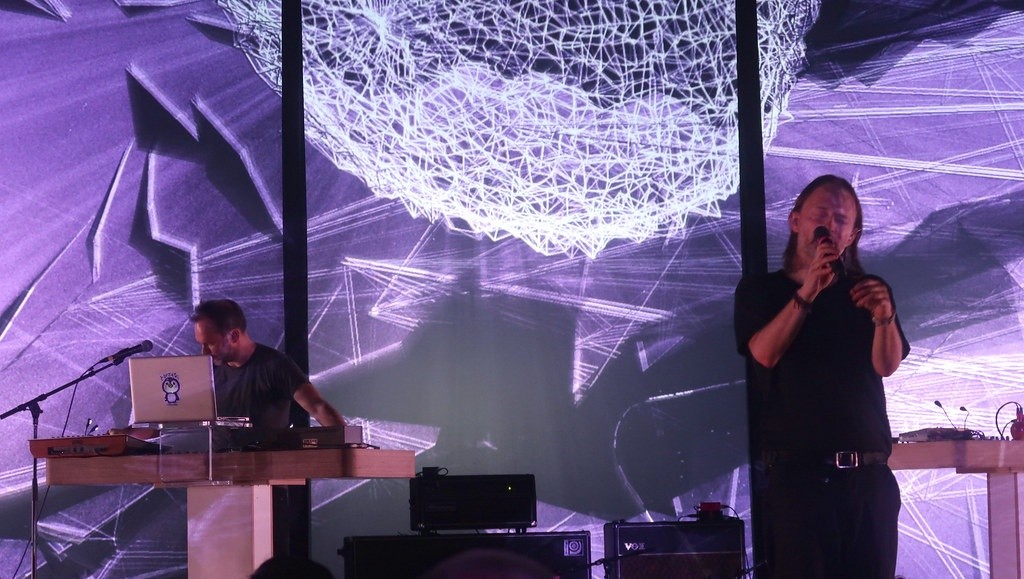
[100,340,153,362]
[935,401,958,432]
[813,226,847,283]
[960,407,970,430]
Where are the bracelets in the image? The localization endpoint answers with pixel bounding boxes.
[871,309,897,326]
[792,292,810,310]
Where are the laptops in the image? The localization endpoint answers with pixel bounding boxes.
[128,356,250,423]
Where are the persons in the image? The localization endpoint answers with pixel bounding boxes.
[107,297,349,556]
[734,173,911,579]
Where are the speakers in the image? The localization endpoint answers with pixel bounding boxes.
[409,475,538,531]
[343,530,591,579]
[602,523,746,579]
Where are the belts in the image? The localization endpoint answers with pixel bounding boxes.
[754,451,888,469]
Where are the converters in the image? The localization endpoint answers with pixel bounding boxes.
[422,466,440,477]
[695,502,725,523]
